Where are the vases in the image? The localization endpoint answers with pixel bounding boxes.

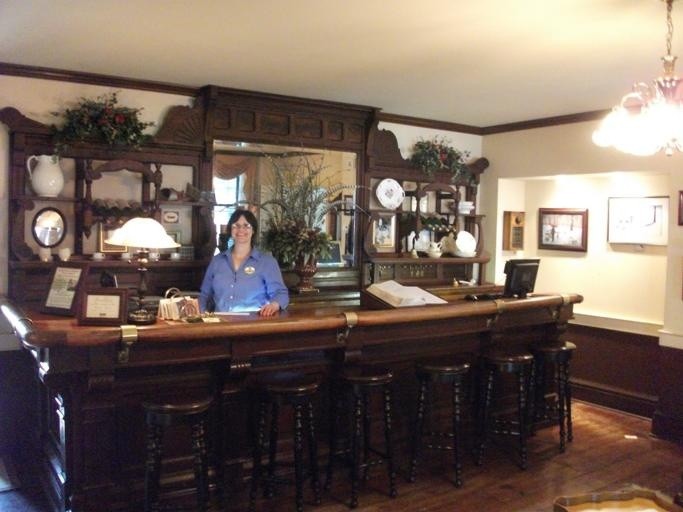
[292,252,321,293]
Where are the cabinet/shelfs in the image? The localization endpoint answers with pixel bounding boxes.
[0,92,206,269]
[360,125,489,265]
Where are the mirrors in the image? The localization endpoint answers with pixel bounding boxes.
[210,137,358,269]
[32,206,67,248]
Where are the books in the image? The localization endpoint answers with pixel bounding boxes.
[367,280,448,307]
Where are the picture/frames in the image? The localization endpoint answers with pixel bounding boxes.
[158,230,181,257]
[371,212,395,249]
[76,286,129,326]
[40,260,90,318]
[343,194,353,216]
[538,207,588,253]
[404,228,432,252]
[409,191,429,214]
[99,221,128,254]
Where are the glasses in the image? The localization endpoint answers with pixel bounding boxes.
[230,221,252,230]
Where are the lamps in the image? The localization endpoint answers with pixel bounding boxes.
[591,0,683,158]
[103,216,181,327]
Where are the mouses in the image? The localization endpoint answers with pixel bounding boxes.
[464,294,478,300]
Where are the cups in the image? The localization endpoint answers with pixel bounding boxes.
[170,252,180,258]
[148,252,160,258]
[38,246,52,262]
[120,253,131,258]
[92,252,106,258]
[56,247,71,262]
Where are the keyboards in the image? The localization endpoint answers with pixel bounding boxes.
[478,294,504,300]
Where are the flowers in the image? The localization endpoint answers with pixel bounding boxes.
[235,146,373,264]
[50,88,155,165]
[407,134,477,186]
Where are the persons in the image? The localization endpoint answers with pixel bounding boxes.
[198,210,290,316]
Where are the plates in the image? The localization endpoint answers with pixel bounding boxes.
[375,178,405,209]
[128,310,156,324]
[456,231,476,252]
[452,252,479,257]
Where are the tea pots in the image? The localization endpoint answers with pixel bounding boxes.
[426,240,444,258]
[27,153,65,199]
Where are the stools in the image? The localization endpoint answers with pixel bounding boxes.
[407,355,471,490]
[471,348,534,472]
[323,366,398,508]
[526,339,577,453]
[250,373,323,511]
[140,381,219,512]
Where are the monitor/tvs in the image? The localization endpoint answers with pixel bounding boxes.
[504,259,540,299]
